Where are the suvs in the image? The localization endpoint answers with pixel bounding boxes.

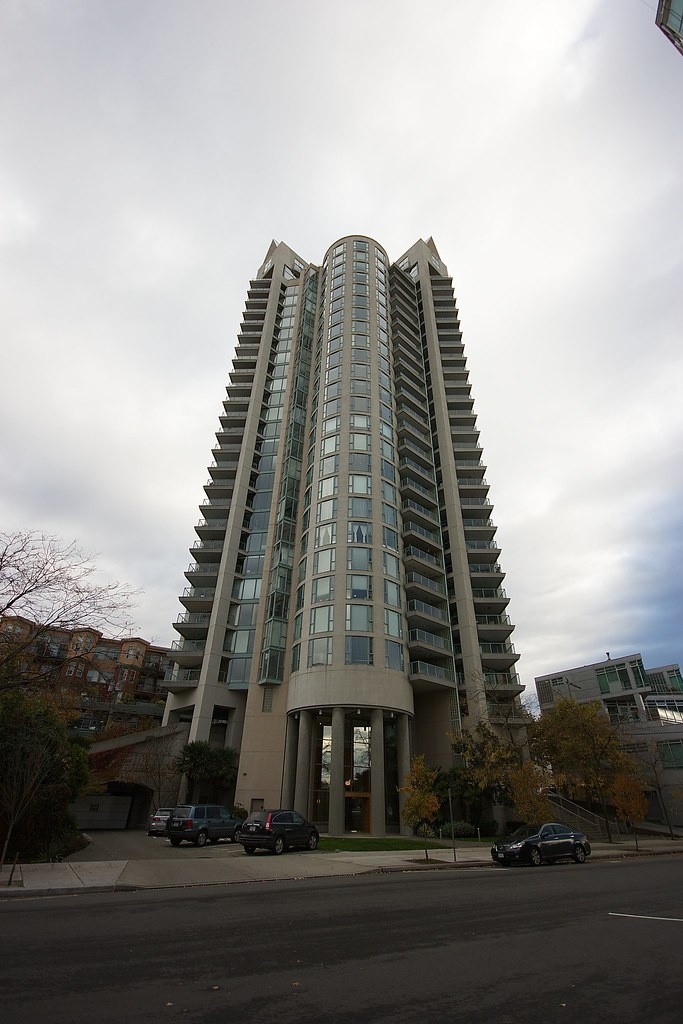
[239,809,319,856]
[165,803,242,847]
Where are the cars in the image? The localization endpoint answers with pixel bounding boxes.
[491,822,591,867]
[148,808,174,836]
